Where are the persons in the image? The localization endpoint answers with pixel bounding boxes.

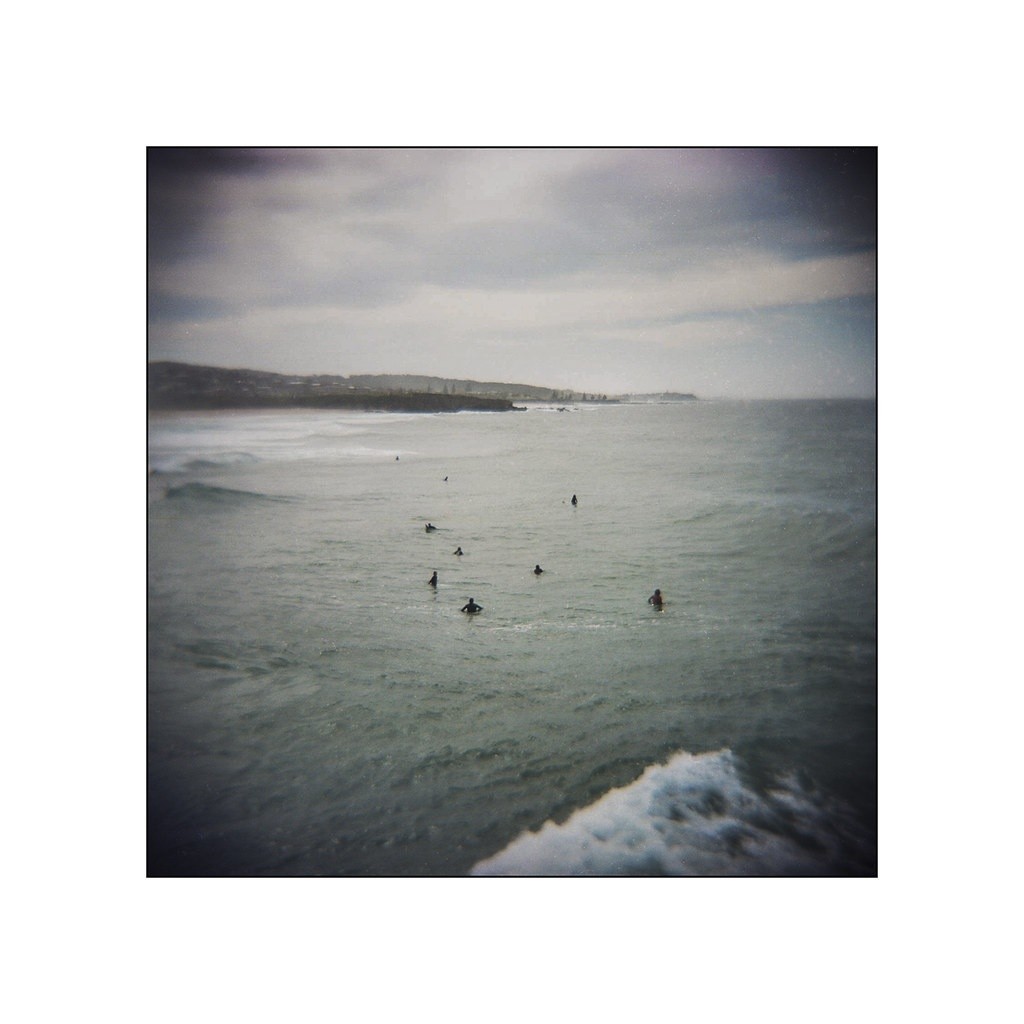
[571,495,578,506]
[534,564,542,574]
[425,523,436,529]
[454,547,464,555]
[461,597,483,613]
[428,571,437,586]
[647,589,663,606]
